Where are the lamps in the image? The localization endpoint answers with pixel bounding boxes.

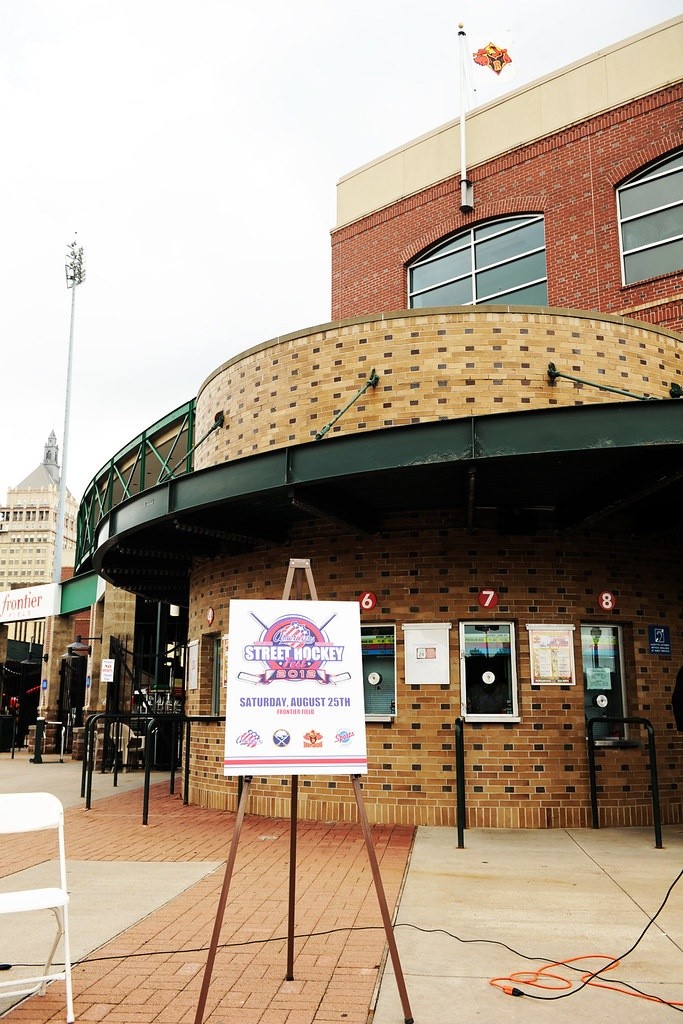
[60,645,91,657]
[66,633,102,647]
[19,651,47,664]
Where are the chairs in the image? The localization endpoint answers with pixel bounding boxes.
[0,792,75,1023]
[109,722,146,774]
